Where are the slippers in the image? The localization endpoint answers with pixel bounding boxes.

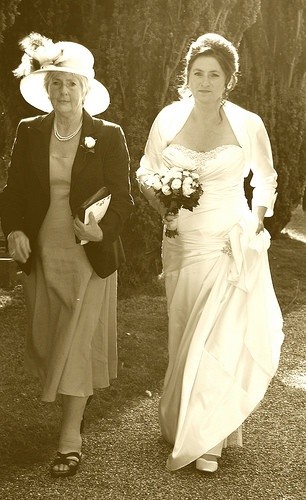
[80,395,92,434]
[50,451,82,477]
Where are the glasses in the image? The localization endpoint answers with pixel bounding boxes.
[49,79,82,90]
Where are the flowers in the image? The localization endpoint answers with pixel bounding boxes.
[80,136,97,162]
[145,166,203,238]
[12,30,64,78]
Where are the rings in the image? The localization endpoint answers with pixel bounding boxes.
[11,251,15,256]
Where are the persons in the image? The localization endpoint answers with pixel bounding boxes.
[0,31,134,478]
[136,32,285,473]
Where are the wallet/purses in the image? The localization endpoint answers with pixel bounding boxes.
[75,186,112,244]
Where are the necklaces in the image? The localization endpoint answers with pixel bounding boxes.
[54,116,84,141]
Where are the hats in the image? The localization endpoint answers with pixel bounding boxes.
[11,31,111,116]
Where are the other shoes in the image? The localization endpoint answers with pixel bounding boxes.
[196,454,218,472]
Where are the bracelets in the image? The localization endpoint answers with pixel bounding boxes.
[7,231,12,240]
